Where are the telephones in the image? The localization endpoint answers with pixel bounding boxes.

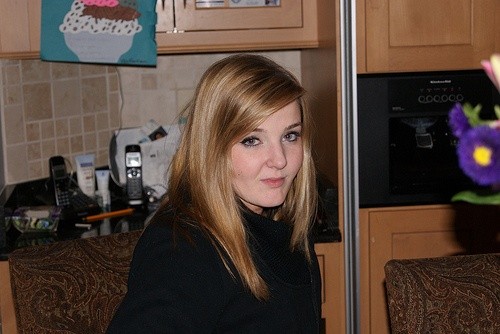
[49,156,71,208]
[125,145,144,205]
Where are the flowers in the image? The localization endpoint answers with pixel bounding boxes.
[447,53,500,206]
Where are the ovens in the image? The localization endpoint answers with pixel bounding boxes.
[357,67,500,209]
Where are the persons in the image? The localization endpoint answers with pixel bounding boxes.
[108,52,323,333]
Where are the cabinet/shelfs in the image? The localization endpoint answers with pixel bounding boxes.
[0,0,500,334]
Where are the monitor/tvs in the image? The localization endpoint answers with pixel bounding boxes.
[358,68,500,208]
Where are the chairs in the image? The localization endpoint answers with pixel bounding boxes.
[385,252,500,334]
[8,230,142,334]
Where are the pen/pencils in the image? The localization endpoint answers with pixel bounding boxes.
[82,208,134,221]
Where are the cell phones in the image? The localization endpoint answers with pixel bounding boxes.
[50,155,70,208]
[125,144,143,204]
